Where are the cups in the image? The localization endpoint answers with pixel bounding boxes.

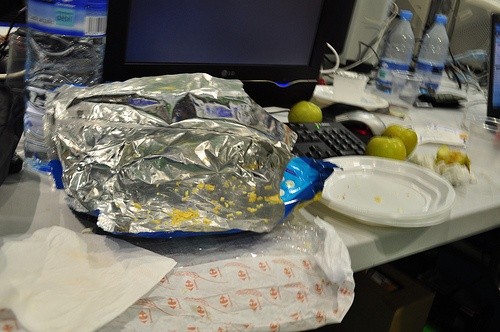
[332,70,369,104]
[0,68,27,184]
[465,116,500,167]
[389,69,425,119]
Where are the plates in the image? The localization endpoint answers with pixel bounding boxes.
[319,155,456,228]
[313,84,390,112]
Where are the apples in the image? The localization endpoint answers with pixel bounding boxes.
[288,100,322,122]
[365,136,406,160]
[384,126,418,159]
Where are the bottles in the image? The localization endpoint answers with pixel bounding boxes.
[23,0,109,161]
[413,14,451,96]
[373,9,417,91]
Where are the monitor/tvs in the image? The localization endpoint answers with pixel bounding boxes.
[105,0,356,108]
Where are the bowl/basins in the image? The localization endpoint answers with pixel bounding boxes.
[38,147,332,242]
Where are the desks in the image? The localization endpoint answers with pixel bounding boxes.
[0,70,500,332]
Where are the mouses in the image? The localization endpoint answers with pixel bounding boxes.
[336,110,387,140]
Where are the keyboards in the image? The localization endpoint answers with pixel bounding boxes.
[280,122,369,160]
